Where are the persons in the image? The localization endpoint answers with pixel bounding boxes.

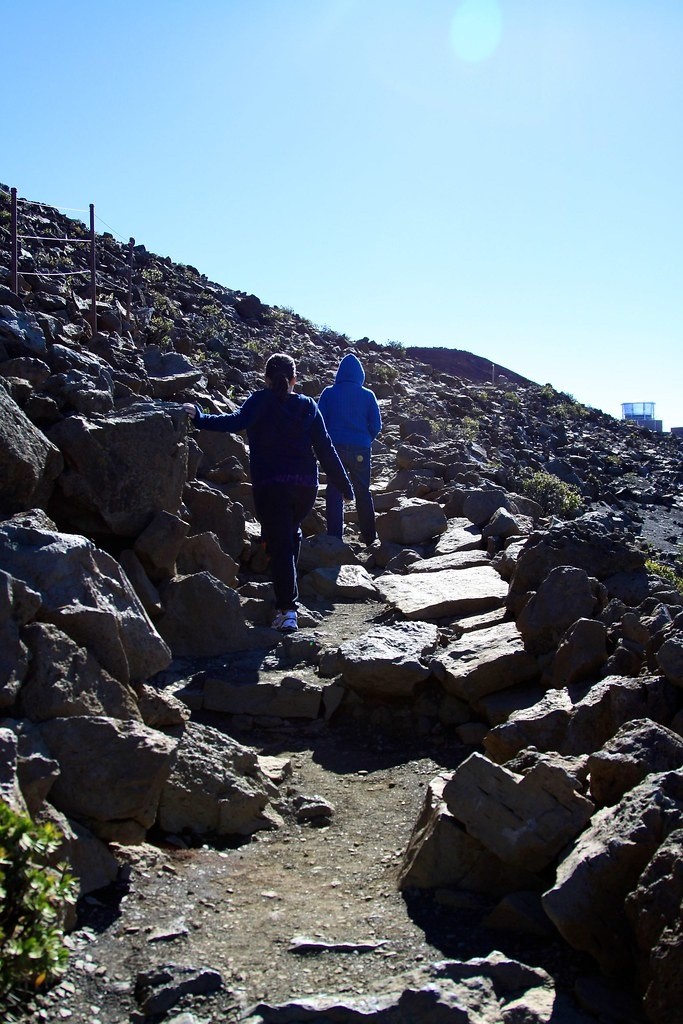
[318,353,382,545]
[183,353,354,631]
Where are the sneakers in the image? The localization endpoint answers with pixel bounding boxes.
[271,609,299,632]
